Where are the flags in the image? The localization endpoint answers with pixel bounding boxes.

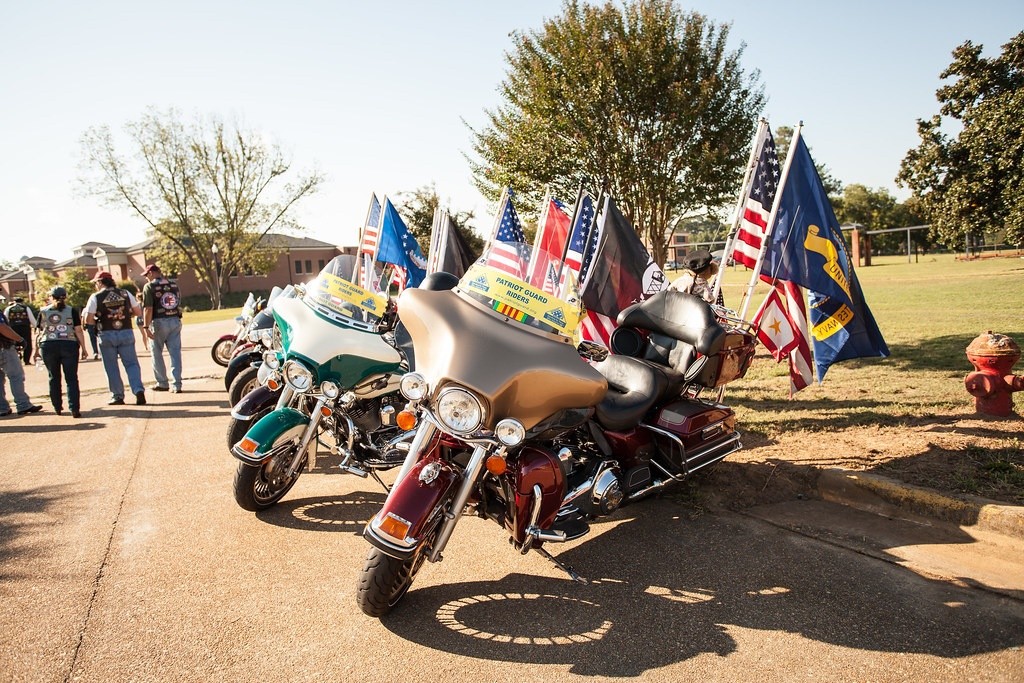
[529,188,670,367]
[696,279,728,359]
[729,128,814,398]
[357,194,428,307]
[482,189,531,281]
[761,132,890,385]
[426,209,478,280]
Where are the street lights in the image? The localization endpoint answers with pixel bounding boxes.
[212,244,229,310]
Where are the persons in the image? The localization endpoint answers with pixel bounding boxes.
[0,310,42,415]
[140,264,183,393]
[3,297,37,365]
[84,271,146,406]
[34,287,89,418]
[136,293,150,350]
[81,308,99,360]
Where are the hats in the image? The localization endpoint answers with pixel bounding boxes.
[14,298,24,301]
[683,250,712,274]
[48,286,66,298]
[89,271,112,282]
[140,264,161,276]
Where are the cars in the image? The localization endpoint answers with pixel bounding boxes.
[663,259,683,271]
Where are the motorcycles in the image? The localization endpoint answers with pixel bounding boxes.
[210,248,462,513]
[356,248,762,616]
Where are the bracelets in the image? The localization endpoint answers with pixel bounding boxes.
[143,326,149,329]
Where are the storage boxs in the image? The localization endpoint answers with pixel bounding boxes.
[692,325,755,388]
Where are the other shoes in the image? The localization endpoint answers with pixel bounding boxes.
[108,398,124,404]
[172,389,181,393]
[72,410,82,418]
[0,408,12,416]
[18,405,42,415]
[56,410,61,415]
[151,385,169,391]
[136,392,146,404]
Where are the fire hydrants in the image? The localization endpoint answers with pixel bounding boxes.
[963,330,1024,416]
